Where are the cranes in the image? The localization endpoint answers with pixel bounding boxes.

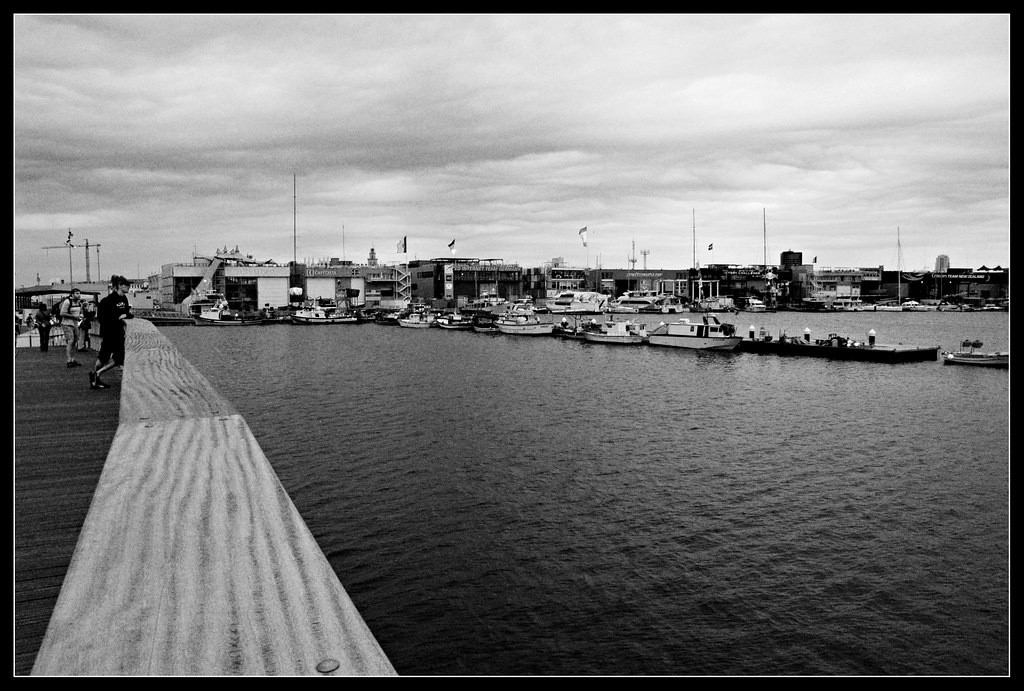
[39,238,102,283]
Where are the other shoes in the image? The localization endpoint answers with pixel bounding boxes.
[67,359,78,367]
[96,379,111,389]
[89,370,99,388]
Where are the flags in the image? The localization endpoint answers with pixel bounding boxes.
[812,256,817,263]
[396,236,407,253]
[708,244,713,250]
[448,239,455,252]
[579,227,587,247]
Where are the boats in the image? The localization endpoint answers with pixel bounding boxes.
[494,298,555,337]
[534,287,737,313]
[744,296,765,312]
[648,316,743,352]
[187,278,500,334]
[828,295,1009,313]
[552,316,649,344]
[940,339,1010,366]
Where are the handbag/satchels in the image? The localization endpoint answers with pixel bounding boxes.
[49,319,55,326]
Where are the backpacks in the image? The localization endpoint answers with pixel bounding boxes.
[52,297,71,320]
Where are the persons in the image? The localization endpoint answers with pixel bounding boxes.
[27,313,33,328]
[36,303,51,352]
[50,288,96,367]
[89,275,134,388]
[15,311,23,346]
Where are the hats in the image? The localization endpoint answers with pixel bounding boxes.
[116,275,134,287]
